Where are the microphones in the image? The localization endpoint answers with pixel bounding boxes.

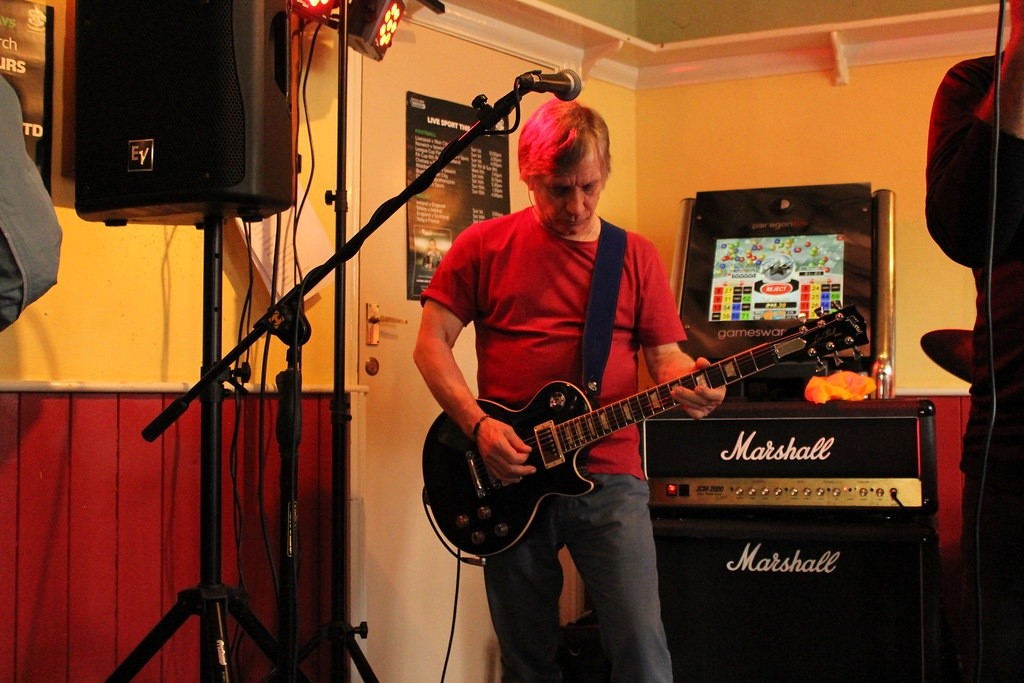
[520,69,582,101]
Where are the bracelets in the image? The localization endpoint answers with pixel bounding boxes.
[470,414,490,441]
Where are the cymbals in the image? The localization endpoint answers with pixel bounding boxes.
[919,328,976,382]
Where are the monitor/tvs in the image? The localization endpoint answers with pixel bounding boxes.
[709,233,844,321]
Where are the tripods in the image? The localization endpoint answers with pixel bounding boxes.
[107,212,309,683]
[256,0,381,683]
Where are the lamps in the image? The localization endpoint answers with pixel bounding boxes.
[291,0,336,26]
[338,0,406,62]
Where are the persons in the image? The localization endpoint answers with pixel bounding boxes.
[921,0,1023,683]
[411,93,729,682]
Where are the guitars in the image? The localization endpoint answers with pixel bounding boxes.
[420,301,872,555]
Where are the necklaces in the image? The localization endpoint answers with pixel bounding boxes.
[584,213,598,243]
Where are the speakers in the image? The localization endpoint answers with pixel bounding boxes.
[649,519,938,683]
[72,0,296,226]
